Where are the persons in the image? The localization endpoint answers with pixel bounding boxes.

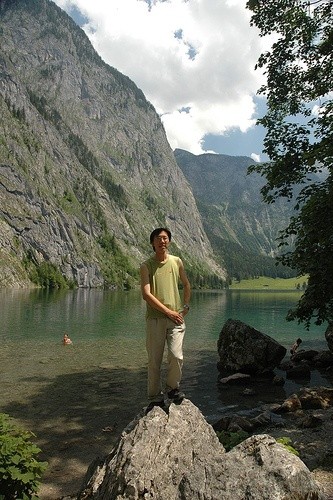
[290,337,302,359]
[63,333,73,345]
[140,227,191,414]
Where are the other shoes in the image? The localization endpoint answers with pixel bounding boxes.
[144,400,165,415]
[168,389,184,401]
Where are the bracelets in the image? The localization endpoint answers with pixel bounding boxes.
[182,304,190,311]
